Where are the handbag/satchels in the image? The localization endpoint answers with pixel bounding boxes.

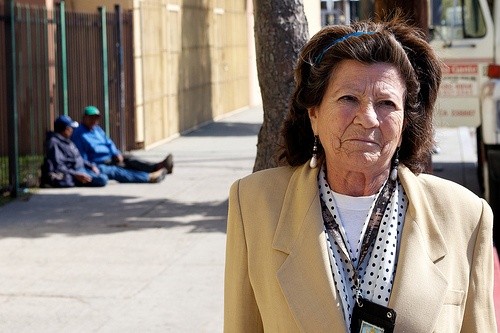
[40,159,75,187]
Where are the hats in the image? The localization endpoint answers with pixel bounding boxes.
[85,105,100,116]
[55,115,79,133]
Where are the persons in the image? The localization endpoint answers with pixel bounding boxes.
[44,106,173,188]
[223,9,496,333]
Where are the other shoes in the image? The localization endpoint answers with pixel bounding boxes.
[149,168,167,182]
[154,154,172,173]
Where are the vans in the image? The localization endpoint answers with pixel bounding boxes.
[425,0,500,245]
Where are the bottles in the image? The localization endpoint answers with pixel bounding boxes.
[479,65,500,144]
